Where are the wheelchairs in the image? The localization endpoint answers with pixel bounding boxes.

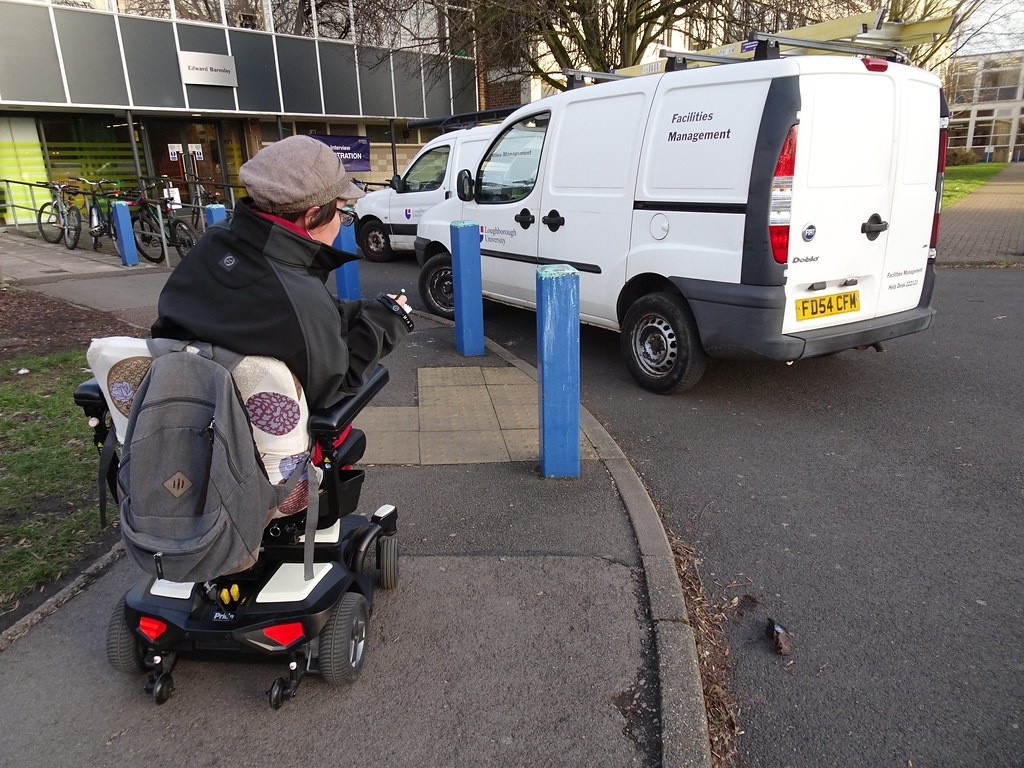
[73,335,399,710]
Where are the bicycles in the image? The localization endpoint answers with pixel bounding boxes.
[190,173,233,230]
[121,181,199,263]
[36,181,82,250]
[68,176,123,257]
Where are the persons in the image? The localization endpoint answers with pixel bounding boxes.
[149,134,414,473]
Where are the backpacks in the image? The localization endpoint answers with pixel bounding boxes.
[97,337,318,583]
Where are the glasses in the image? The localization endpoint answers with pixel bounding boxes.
[320,205,357,227]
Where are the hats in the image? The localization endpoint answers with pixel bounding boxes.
[238,135,366,213]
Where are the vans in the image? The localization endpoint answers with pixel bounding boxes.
[354,107,520,262]
[413,15,950,393]
